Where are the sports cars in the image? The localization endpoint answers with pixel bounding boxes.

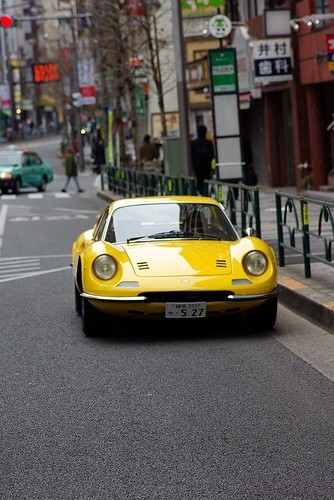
[71,196,281,340]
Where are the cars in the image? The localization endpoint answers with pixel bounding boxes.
[0,145,54,195]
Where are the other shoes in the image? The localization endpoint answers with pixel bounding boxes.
[61,190,65,192]
[78,189,83,192]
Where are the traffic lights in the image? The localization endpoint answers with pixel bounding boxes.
[0,15,16,27]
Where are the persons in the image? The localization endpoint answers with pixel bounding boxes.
[190,125,214,195]
[138,135,158,172]
[61,147,84,192]
[91,137,107,176]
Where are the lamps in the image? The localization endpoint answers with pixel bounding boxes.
[291,13,334,30]
